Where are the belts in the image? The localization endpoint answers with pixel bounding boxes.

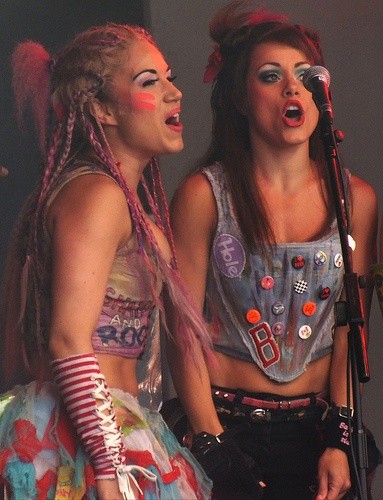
[211,389,329,420]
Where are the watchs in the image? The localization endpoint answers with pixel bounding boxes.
[326,405,358,427]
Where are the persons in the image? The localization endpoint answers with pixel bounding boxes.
[170,22,378,500]
[0,24,218,499]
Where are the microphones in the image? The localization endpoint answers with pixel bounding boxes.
[303,66,334,123]
